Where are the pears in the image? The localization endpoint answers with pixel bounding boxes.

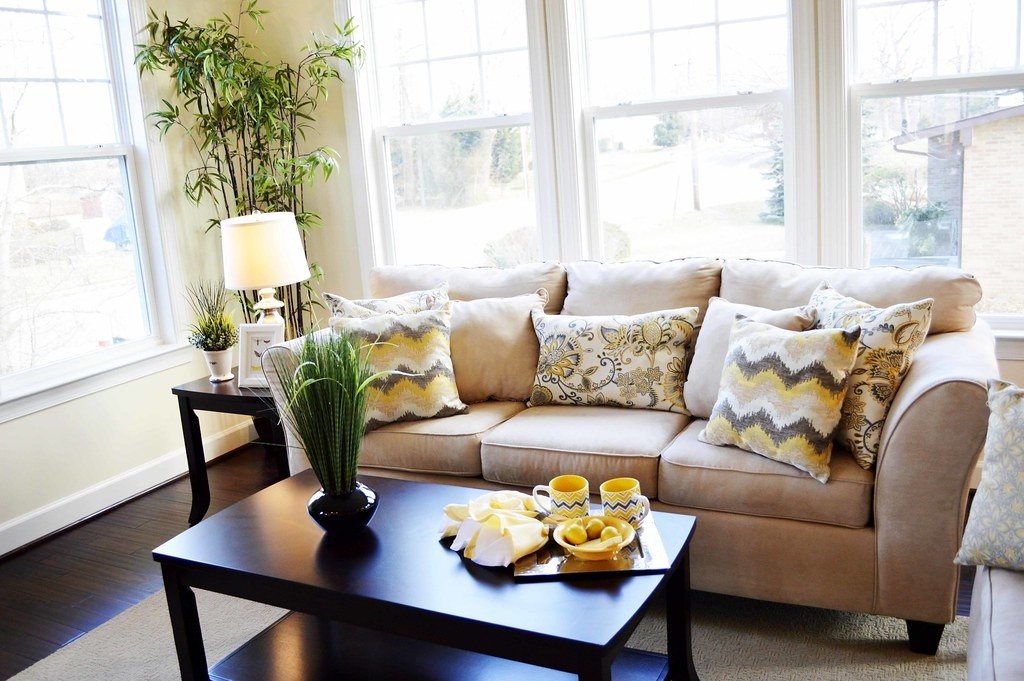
[565,517,621,543]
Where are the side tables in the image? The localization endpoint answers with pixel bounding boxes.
[171,364,288,527]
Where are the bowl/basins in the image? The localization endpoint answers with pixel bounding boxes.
[554,516,636,561]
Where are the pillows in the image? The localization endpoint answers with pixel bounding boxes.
[695,312,861,485]
[682,296,819,418]
[807,278,935,470]
[321,279,470,437]
[449,287,549,405]
[952,377,1024,573]
[526,306,700,417]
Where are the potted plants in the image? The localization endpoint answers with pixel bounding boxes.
[243,317,426,536]
[180,274,241,383]
[132,1,368,461]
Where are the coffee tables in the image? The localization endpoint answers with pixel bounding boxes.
[150,467,699,681]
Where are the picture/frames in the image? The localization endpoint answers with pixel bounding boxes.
[237,323,285,388]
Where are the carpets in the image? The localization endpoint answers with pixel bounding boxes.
[2,588,969,681]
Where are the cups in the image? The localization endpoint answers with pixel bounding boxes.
[533,474,589,521]
[600,478,648,529]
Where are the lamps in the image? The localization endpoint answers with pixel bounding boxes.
[219,211,312,324]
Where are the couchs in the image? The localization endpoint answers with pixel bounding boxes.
[965,566,1024,681]
[261,259,1003,655]
